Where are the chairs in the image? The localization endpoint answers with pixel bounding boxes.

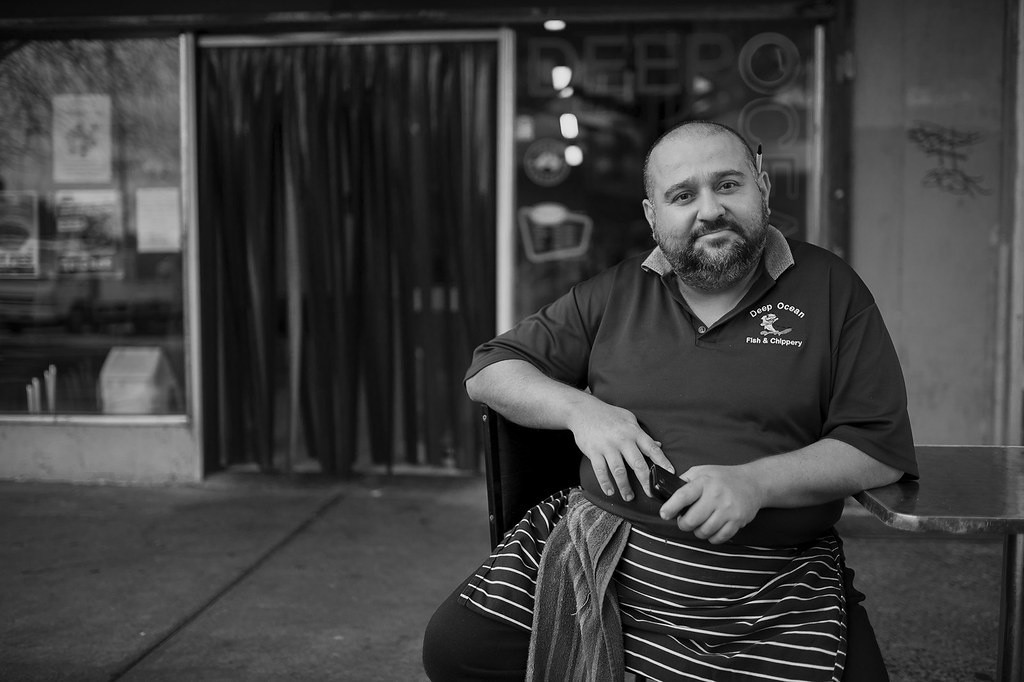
[479,402,582,552]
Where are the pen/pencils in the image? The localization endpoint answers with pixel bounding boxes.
[756,143,762,173]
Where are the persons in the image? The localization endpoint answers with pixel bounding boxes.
[423,120,918,682]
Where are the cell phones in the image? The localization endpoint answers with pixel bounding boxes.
[650,464,698,517]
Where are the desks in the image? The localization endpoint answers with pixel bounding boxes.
[853,446,1023,682]
